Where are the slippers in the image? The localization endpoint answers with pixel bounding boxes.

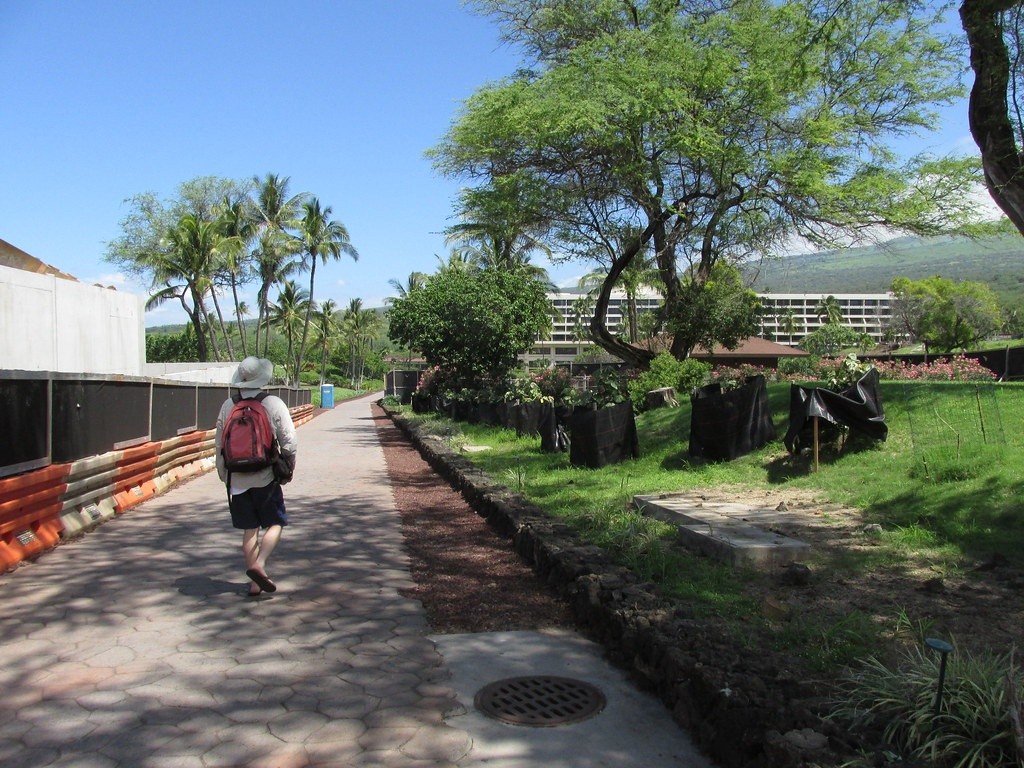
[247,589,262,596]
[246,568,276,593]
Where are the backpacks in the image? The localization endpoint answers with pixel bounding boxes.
[221,391,279,472]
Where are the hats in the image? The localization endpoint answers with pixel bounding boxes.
[231,356,273,388]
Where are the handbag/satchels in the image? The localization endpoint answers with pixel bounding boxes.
[272,454,293,486]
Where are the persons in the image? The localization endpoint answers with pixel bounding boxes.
[214,355,297,596]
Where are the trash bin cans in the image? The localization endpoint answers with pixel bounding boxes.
[321,384,334,409]
